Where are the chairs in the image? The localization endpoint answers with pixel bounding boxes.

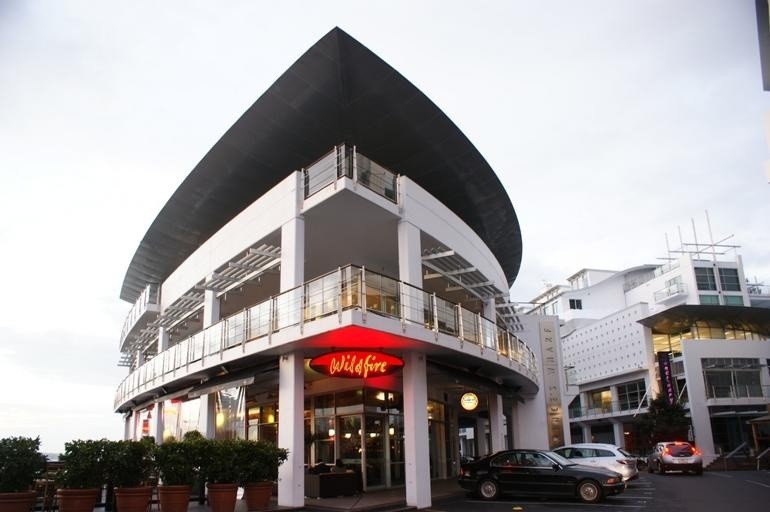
[30,477,55,511]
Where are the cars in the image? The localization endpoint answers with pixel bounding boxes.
[645,440,704,476]
[454,442,642,503]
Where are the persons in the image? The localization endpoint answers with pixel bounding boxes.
[332,459,346,473]
[309,459,329,474]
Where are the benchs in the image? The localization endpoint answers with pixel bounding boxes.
[306,467,355,500]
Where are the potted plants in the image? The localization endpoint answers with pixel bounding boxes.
[54,438,113,511]
[151,440,199,511]
[106,439,155,512]
[198,437,249,511]
[239,438,290,511]
[0,434,49,512]
